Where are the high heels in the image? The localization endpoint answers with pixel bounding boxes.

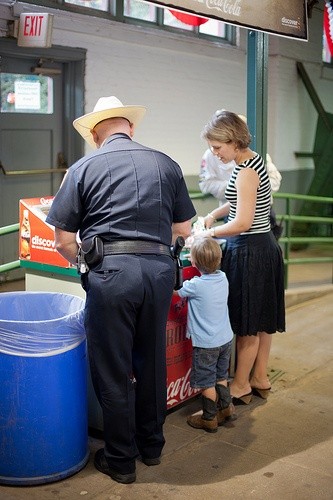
[233,391,252,405]
[255,387,270,400]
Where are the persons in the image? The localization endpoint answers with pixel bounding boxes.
[194,107,287,404]
[166,238,238,433]
[198,113,284,242]
[45,92,196,484]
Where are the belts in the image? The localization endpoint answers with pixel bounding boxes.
[102,241,172,257]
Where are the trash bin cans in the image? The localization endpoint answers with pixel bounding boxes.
[0,291,92,488]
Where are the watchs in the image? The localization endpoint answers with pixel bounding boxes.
[210,227,216,237]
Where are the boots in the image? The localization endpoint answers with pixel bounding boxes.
[216,383,231,421]
[186,394,217,432]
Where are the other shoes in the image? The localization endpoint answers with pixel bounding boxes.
[143,453,160,466]
[94,448,136,484]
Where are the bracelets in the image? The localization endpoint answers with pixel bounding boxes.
[208,213,217,222]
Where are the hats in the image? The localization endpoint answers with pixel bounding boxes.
[73,96,146,147]
